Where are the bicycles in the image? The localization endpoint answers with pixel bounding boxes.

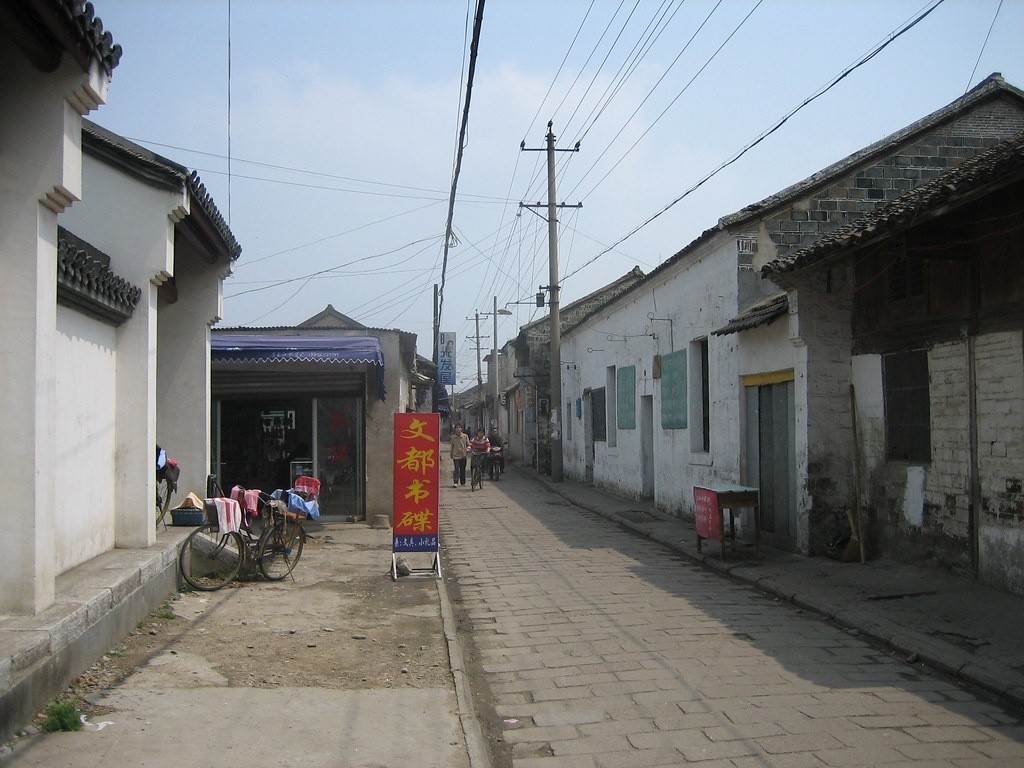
[156,473,174,531]
[179,484,314,592]
[466,447,491,493]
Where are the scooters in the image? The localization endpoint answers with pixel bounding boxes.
[486,441,508,481]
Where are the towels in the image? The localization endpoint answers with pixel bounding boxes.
[230,486,259,515]
[203,498,242,535]
[262,477,320,522]
[172,491,202,510]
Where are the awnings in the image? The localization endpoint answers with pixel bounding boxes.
[211,336,386,401]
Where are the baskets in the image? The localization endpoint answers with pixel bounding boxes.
[472,452,484,468]
[204,500,220,525]
[171,507,205,526]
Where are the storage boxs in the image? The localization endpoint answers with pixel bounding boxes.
[171,506,203,525]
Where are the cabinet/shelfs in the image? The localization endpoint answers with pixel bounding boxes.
[288,457,314,486]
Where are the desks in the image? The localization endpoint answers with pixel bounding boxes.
[692,482,762,564]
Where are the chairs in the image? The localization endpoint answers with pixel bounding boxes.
[268,475,320,548]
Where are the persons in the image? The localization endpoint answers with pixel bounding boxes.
[489,428,506,474]
[449,424,469,488]
[466,427,470,438]
[467,428,491,480]
[282,442,309,485]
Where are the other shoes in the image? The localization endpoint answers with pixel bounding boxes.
[461,483,466,487]
[501,469,505,474]
[453,483,458,488]
[490,469,493,474]
